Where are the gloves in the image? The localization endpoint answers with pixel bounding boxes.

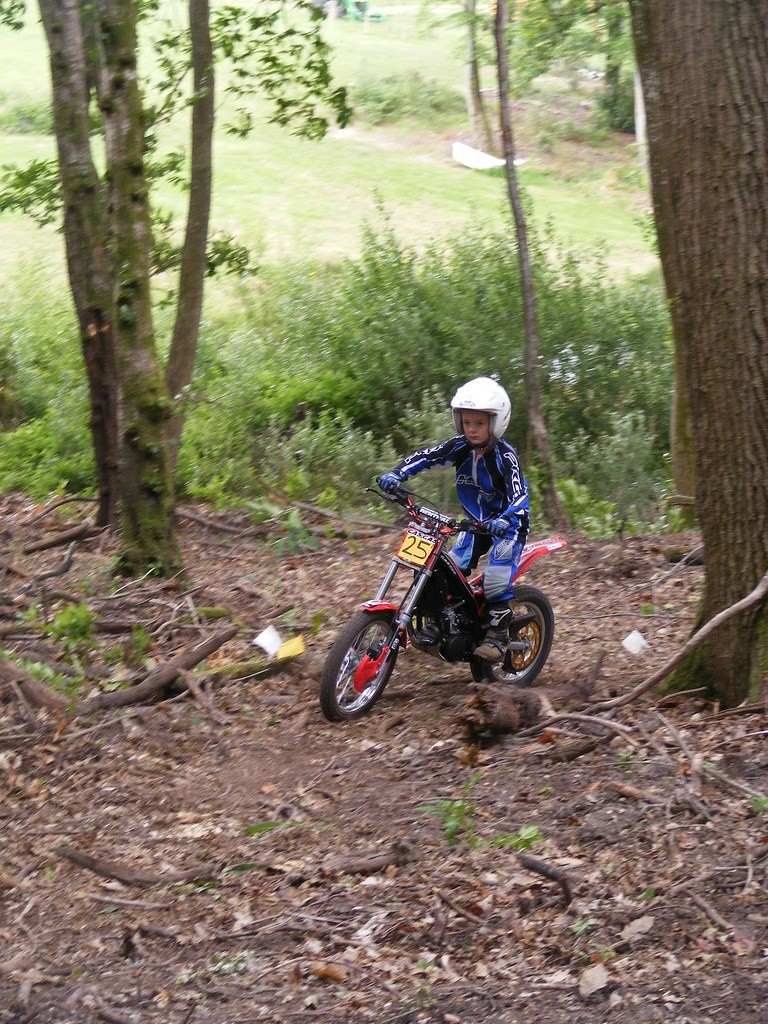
[481,516,510,535]
[378,472,403,494]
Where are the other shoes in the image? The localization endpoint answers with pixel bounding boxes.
[473,627,509,662]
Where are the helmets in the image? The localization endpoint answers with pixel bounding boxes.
[450,377,511,440]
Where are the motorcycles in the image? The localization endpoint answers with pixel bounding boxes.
[320,474,568,723]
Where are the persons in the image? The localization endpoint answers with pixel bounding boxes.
[377,377,530,663]
